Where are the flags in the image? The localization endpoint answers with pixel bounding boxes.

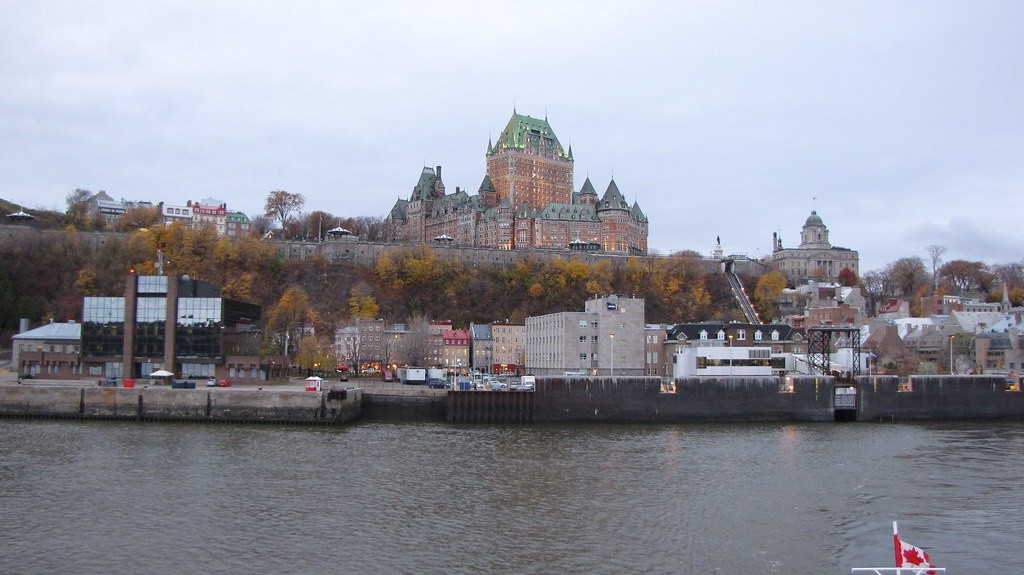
[894,533,936,575]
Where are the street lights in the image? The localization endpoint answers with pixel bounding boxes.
[609,333,615,376]
[729,335,734,378]
[950,336,955,375]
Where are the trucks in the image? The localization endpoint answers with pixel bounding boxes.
[428,368,447,380]
[400,369,425,385]
[521,376,536,388]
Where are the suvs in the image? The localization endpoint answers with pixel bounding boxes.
[427,377,451,388]
[486,381,508,389]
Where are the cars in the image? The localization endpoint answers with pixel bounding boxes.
[510,382,519,390]
[206,378,230,388]
[525,381,535,391]
[383,371,393,383]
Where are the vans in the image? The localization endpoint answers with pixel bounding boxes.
[563,372,586,378]
